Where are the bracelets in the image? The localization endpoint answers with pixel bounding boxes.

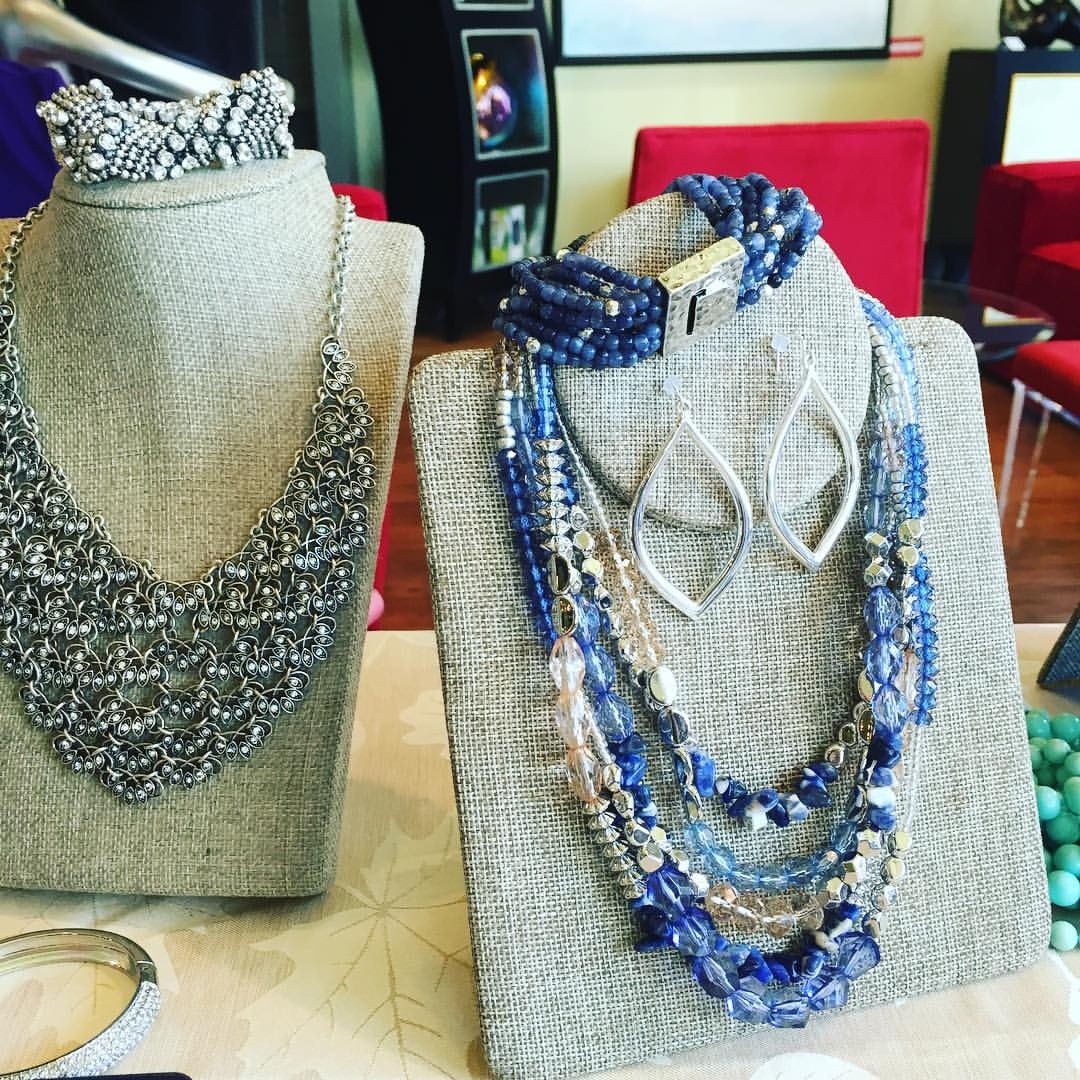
[1,927,160,1080]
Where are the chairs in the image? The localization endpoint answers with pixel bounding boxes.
[997,341,1080,532]
[628,121,932,323]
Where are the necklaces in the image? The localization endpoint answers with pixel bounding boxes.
[494,290,936,1027]
[1,194,375,807]
[36,66,294,184]
[490,171,822,365]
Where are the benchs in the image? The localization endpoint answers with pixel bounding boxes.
[970,159,1080,340]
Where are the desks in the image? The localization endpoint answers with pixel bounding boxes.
[912,279,1055,361]
[3,622,1080,1080]
